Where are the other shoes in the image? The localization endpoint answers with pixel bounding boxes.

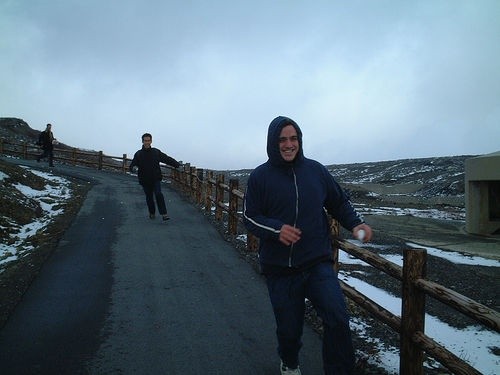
[36,155,40,163]
[150,213,156,220]
[280,359,302,375]
[49,165,55,167]
[163,216,170,221]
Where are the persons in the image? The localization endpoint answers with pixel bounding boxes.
[129,133,180,221]
[37,124,54,167]
[242,115,373,375]
